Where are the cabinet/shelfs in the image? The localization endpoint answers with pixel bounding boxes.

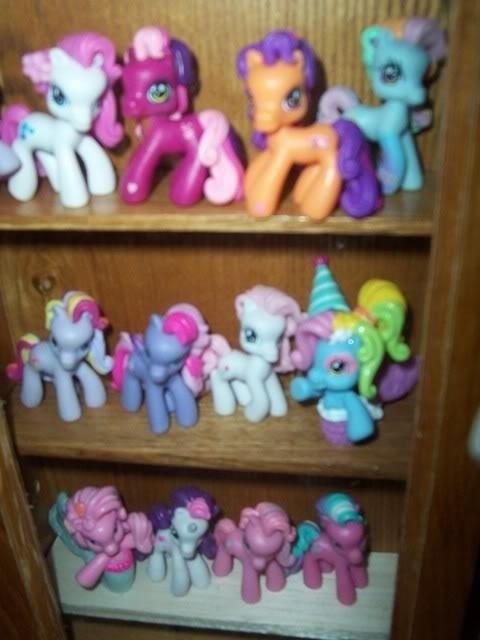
[0,0,480,640]
